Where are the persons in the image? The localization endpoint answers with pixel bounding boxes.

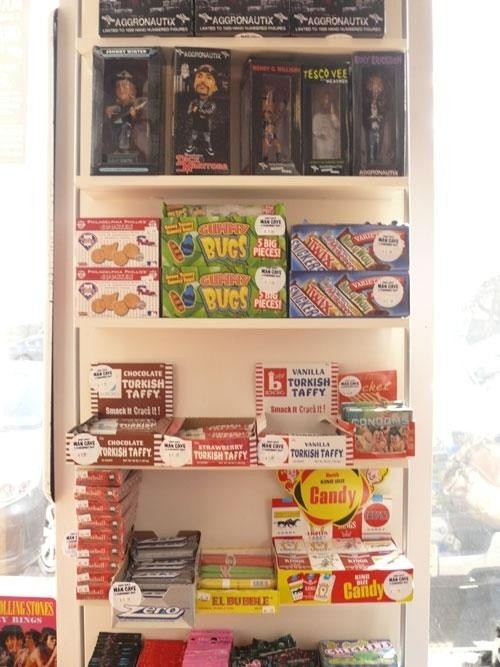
[355,424,410,457]
[365,76,386,165]
[0,624,57,667]
[308,88,342,160]
[105,69,149,155]
[185,63,218,160]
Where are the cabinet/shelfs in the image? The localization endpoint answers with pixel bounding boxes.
[51,0,434,667]
